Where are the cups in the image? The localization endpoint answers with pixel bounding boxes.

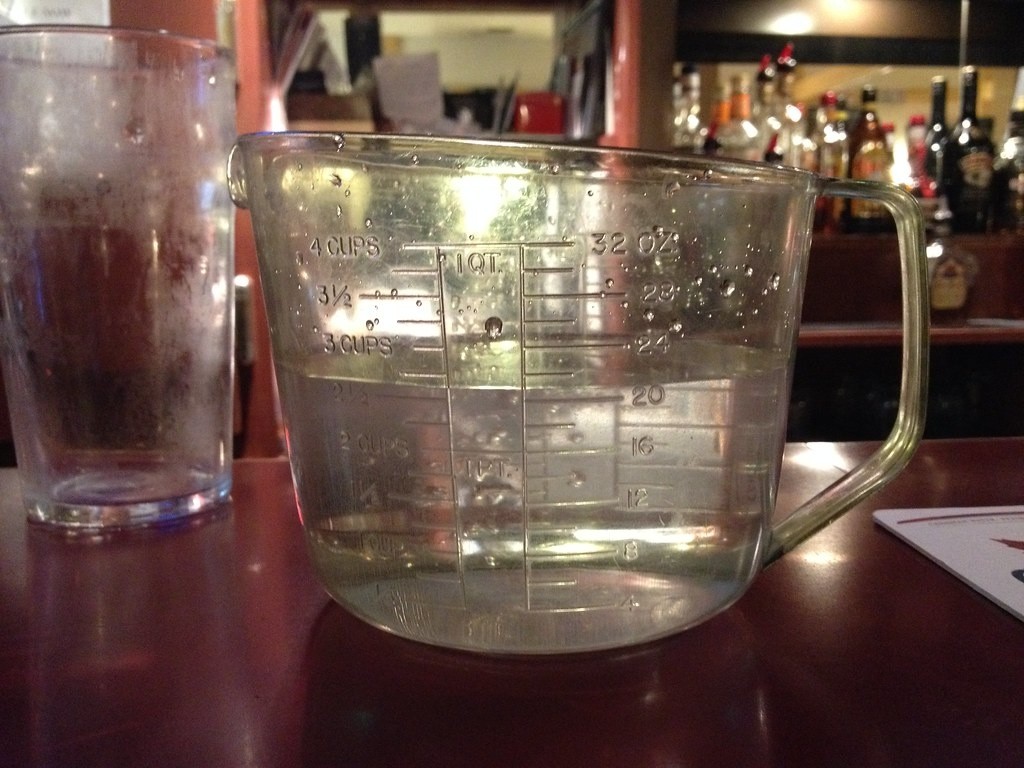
[238,129,925,654]
[1,23,234,530]
[515,92,563,136]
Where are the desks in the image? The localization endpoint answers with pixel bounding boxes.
[0,433,1024,768]
[797,315,1024,347]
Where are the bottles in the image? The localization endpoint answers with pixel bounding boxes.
[923,212,974,327]
[551,55,577,103]
[568,53,603,143]
[672,51,1024,234]
